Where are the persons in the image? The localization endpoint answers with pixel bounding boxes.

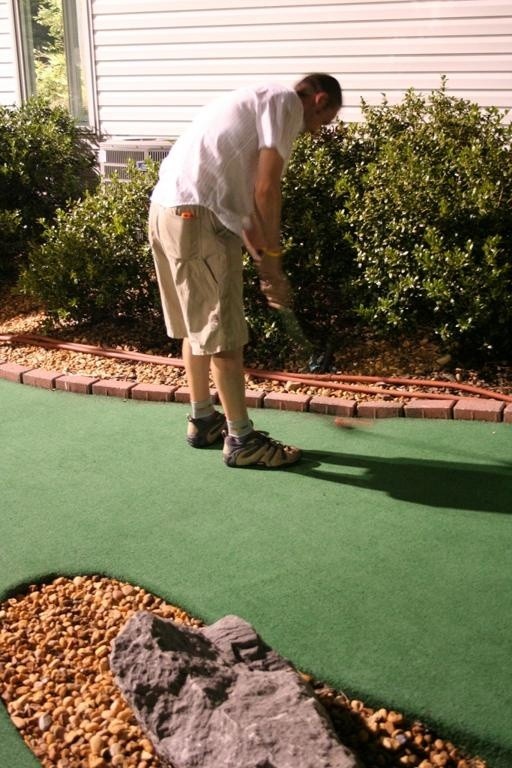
[149,74,342,468]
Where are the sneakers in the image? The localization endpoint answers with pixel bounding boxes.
[186,410,254,447]
[221,426,303,468]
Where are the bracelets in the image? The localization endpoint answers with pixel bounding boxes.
[263,247,281,260]
[250,249,265,263]
